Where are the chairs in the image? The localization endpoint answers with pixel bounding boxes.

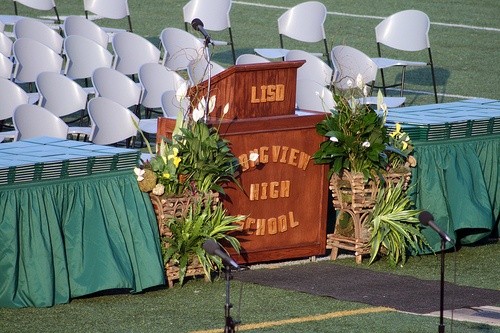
[0,1,439,145]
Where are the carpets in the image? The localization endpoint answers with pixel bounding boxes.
[231,260,500,326]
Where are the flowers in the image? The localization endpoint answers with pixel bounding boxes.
[315,39,415,186]
[133,72,259,200]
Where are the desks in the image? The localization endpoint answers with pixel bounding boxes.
[380,98,500,256]
[0,134,168,307]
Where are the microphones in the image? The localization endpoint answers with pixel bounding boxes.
[420,211,452,241]
[202,238,240,269]
[190,18,214,43]
